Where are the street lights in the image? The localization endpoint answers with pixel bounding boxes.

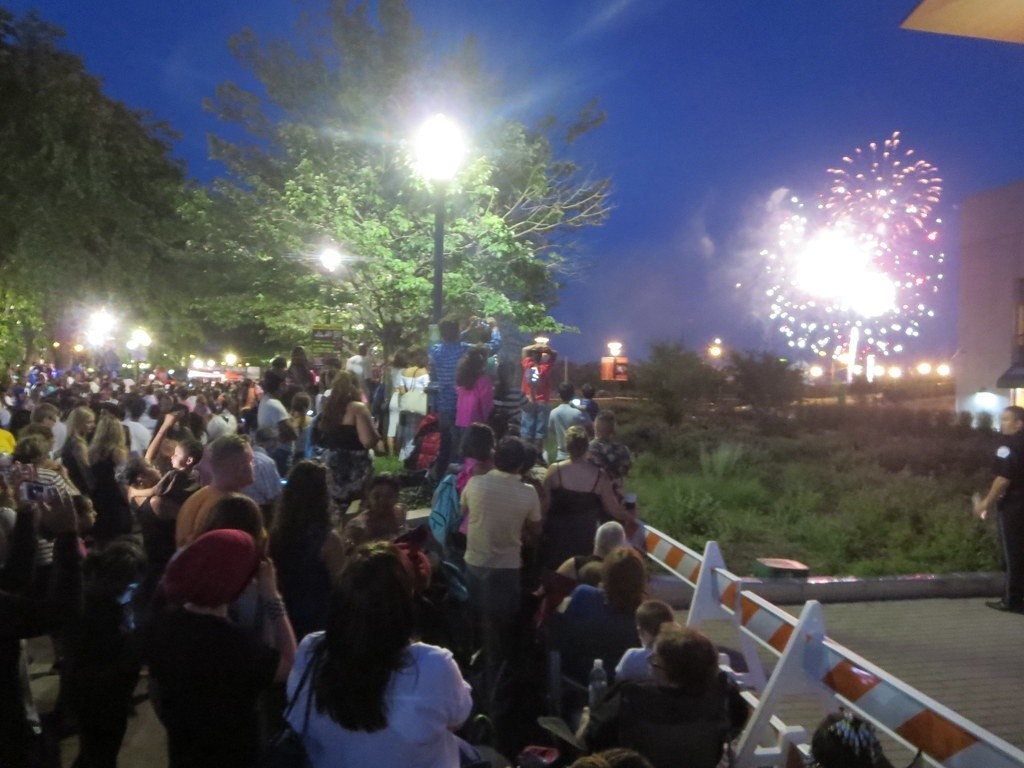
[126,328,152,384]
[706,337,724,372]
[601,341,629,407]
[224,352,248,380]
[410,109,475,332]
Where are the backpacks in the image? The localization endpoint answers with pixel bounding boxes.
[427,474,465,547]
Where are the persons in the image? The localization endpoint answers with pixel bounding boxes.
[0,313,893,768]
[975,406,1024,614]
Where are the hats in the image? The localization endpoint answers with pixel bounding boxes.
[149,528,260,609]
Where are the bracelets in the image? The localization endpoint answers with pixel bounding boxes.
[272,594,282,604]
